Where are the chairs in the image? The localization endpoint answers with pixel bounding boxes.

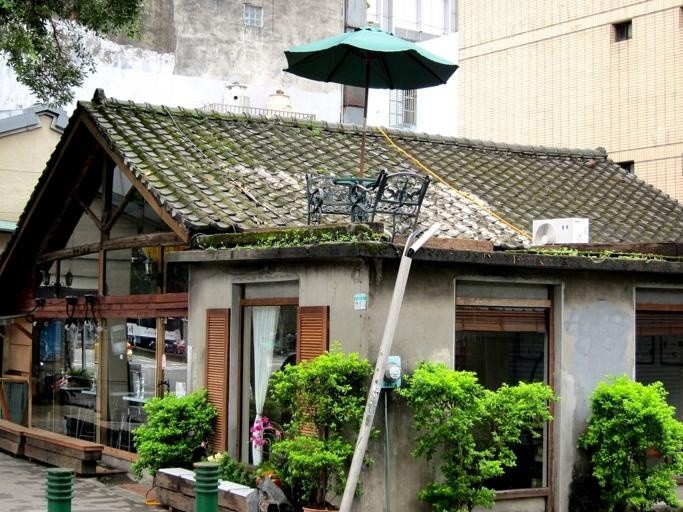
[305,173,431,242]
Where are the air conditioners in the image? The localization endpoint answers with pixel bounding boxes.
[532,217,589,245]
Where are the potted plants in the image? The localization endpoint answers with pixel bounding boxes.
[268,337,380,512]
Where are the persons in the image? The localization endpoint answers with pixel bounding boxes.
[280,333,296,372]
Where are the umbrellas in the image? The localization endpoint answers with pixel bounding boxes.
[282,20,459,178]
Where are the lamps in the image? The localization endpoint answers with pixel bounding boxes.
[60,269,75,293]
[41,270,56,293]
[142,252,162,287]
